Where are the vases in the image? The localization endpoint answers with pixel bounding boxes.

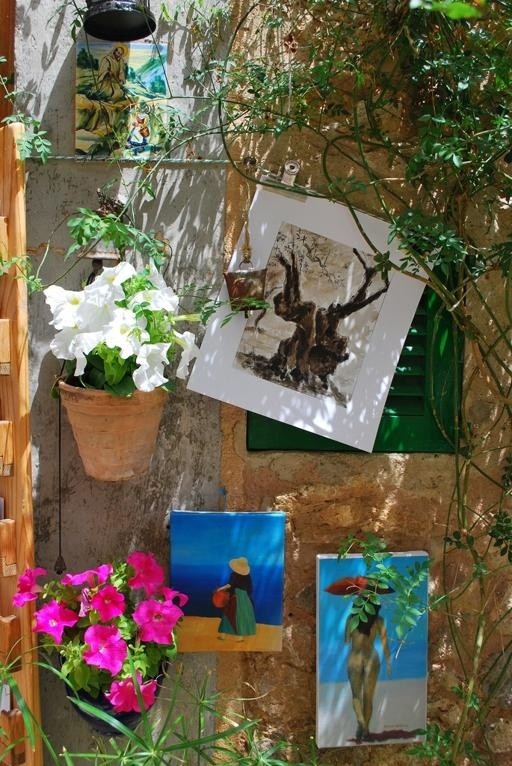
[50,372,175,486]
[54,647,165,738]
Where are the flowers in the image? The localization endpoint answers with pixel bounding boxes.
[9,541,192,722]
[39,204,204,400]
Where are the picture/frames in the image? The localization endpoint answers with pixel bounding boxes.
[180,172,443,456]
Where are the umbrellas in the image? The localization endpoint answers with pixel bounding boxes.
[325,576,396,595]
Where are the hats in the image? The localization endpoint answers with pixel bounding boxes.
[231,558,251,576]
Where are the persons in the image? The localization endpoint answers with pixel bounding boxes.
[98,44,128,100]
[217,557,257,642]
[344,597,393,738]
